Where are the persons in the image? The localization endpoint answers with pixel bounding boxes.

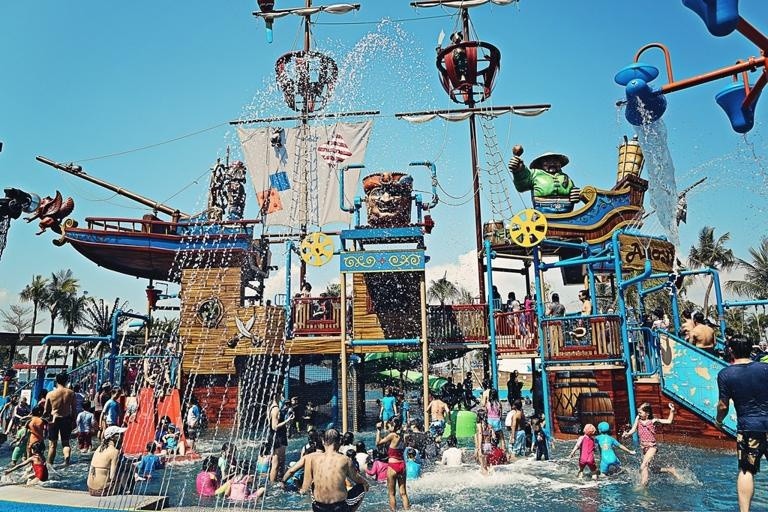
[313,293,331,319]
[1,358,139,496]
[200,390,314,500]
[492,285,592,346]
[300,370,549,512]
[137,396,201,477]
[714,334,768,512]
[362,172,414,226]
[508,152,581,213]
[569,403,675,486]
[639,306,768,367]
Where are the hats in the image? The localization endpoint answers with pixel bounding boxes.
[104,426,128,440]
[598,422,610,432]
[168,424,175,428]
